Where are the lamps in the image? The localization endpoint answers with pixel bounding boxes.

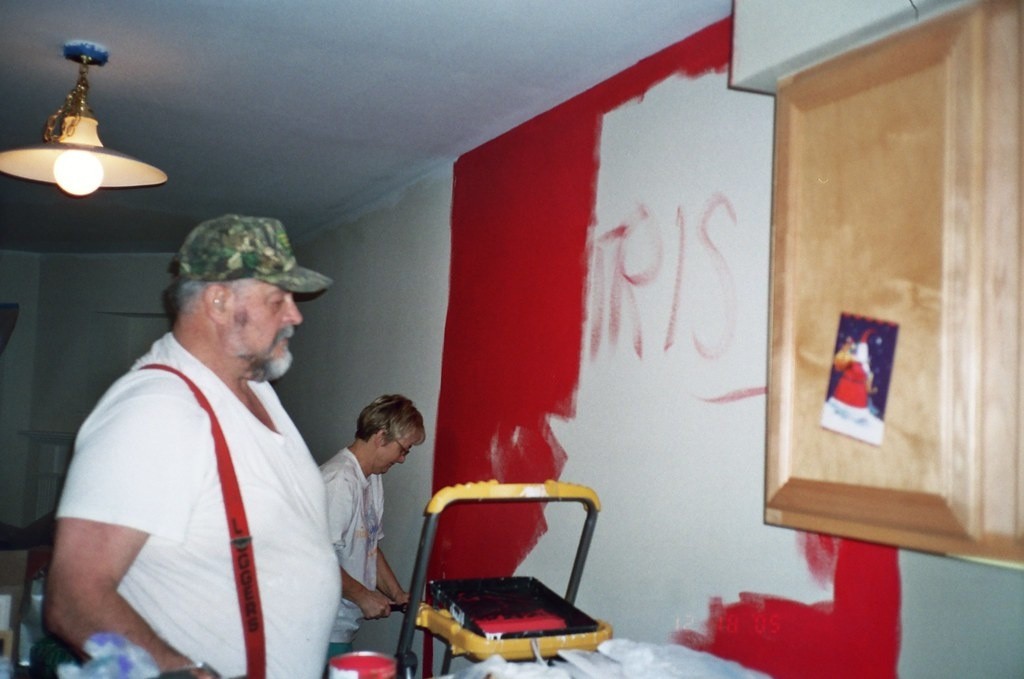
[0,42,167,196]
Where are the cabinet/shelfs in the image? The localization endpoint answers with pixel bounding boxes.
[728,0,1024,564]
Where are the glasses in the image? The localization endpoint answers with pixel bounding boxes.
[395,439,410,455]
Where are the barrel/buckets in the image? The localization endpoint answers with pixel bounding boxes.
[328,651,398,679]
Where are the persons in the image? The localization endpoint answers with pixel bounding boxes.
[318,394,426,666]
[43,213,342,678]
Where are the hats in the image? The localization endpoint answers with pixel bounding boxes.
[174,214,333,302]
